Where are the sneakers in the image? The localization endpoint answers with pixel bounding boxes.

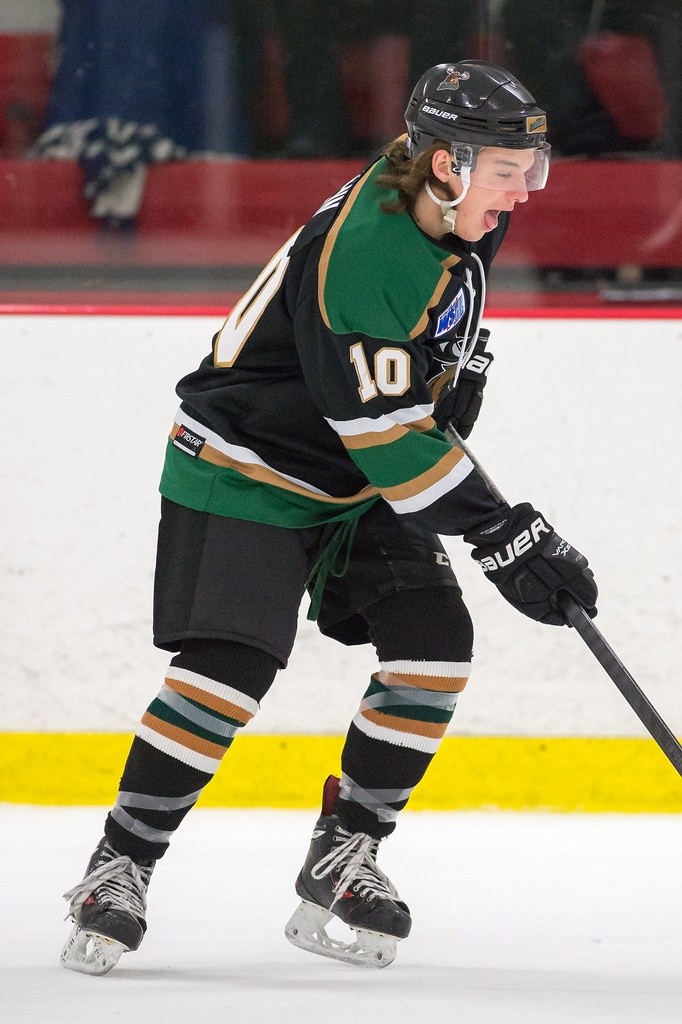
[60,835,156,977]
[282,774,412,968]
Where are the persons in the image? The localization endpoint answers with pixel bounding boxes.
[54,60,602,976]
[55,4,657,154]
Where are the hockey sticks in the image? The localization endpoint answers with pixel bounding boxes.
[437,423,681,777]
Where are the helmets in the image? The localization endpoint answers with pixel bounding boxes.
[404,59,547,174]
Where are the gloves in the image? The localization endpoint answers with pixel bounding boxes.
[463,502,598,626]
[434,329,492,440]
